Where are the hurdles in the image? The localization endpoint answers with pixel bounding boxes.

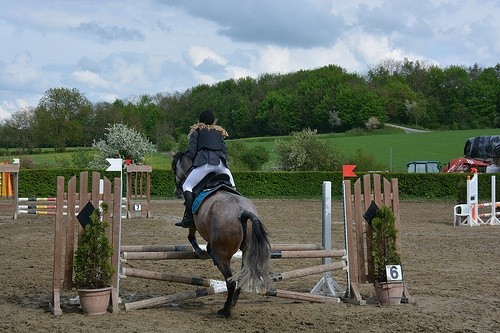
[454,172,500,226]
[50,172,414,318]
[0,163,153,219]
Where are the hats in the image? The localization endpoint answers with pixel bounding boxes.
[199,109,215,125]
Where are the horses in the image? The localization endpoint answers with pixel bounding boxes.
[171,143,278,316]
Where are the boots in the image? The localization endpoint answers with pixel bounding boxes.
[175,190,195,228]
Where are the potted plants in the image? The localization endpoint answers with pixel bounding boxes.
[371,206,404,306]
[72,203,115,315]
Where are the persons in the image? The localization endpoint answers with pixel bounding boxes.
[175,111,236,228]
